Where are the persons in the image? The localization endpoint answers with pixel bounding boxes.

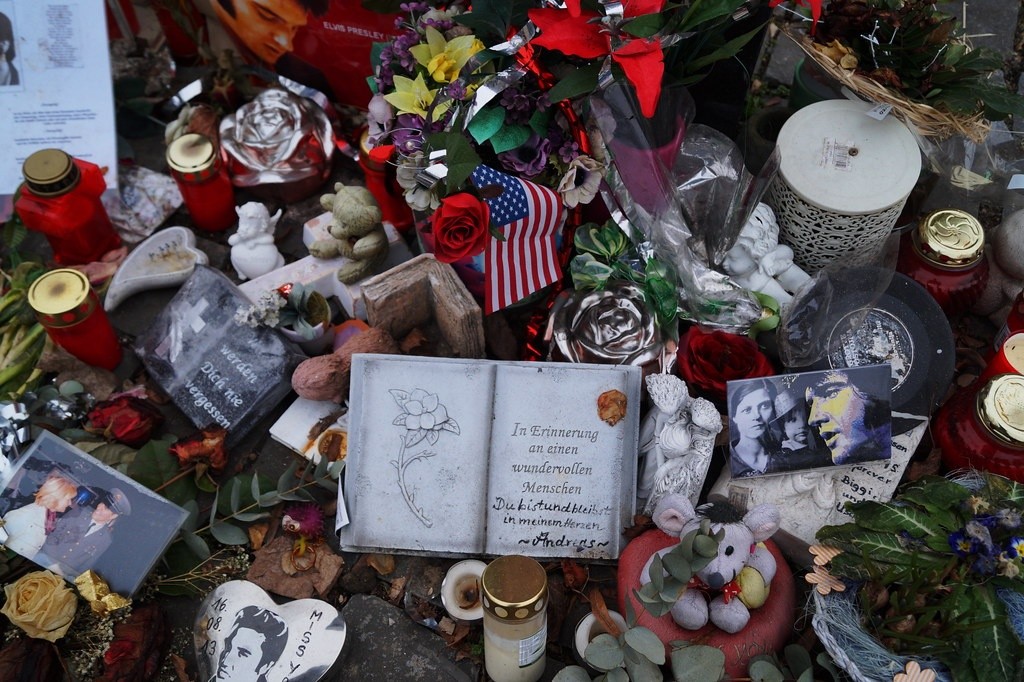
[0,475,78,561]
[213,0,328,67]
[32,486,133,580]
[728,361,891,478]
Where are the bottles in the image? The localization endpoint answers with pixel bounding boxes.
[932,374,1024,487]
[896,208,989,311]
[12,148,113,264]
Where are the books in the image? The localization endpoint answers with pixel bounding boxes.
[337,351,644,564]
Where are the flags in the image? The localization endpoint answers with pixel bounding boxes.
[466,162,567,317]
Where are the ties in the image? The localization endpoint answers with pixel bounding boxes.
[85,522,96,535]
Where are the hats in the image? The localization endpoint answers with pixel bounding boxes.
[101,488,131,517]
[769,388,804,426]
